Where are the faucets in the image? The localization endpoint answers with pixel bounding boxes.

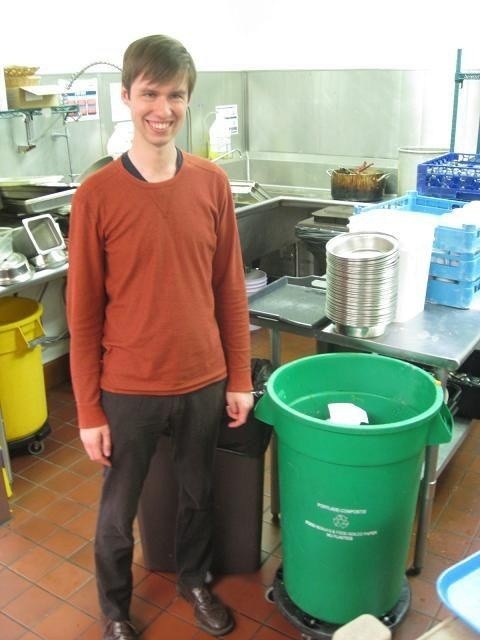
[212,149,242,163]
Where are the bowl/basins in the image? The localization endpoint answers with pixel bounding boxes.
[0,251,36,286]
[324,230,401,339]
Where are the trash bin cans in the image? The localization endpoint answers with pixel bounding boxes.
[135,357,270,574]
[0,295,51,455]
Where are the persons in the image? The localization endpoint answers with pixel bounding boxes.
[65,33,254,640]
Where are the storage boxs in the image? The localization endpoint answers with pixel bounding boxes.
[8,84,63,109]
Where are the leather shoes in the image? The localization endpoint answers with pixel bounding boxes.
[99,612,141,640]
[173,578,239,638]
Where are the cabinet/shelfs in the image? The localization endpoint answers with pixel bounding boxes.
[247,204,480,577]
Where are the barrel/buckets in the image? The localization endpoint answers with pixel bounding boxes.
[0,297,49,442]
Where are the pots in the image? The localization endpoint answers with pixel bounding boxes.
[326,166,392,203]
